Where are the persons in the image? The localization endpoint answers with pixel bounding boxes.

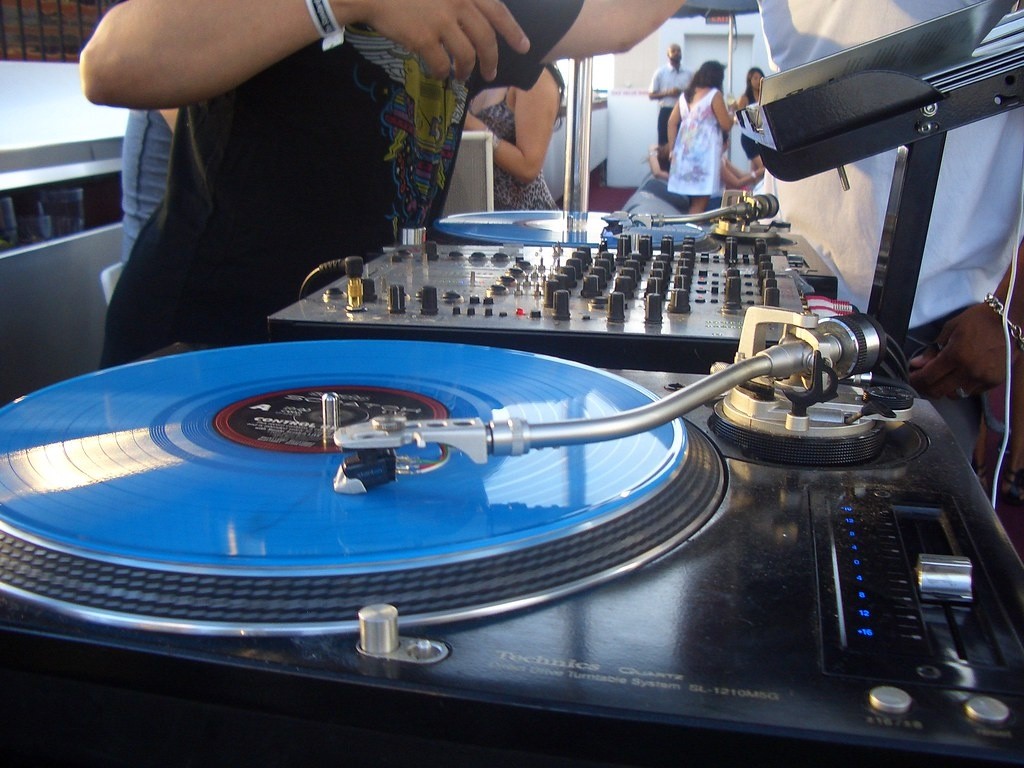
[719,132,765,194]
[972,356,1024,497]
[757,1,1024,469]
[462,61,566,213]
[649,44,693,180]
[737,68,766,169]
[667,61,736,224]
[79,0,687,372]
[122,108,181,268]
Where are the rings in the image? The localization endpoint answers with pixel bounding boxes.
[955,386,968,399]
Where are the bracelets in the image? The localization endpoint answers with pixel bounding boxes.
[984,293,1024,352]
[728,112,735,115]
[485,128,501,152]
[305,0,345,51]
[751,172,756,178]
